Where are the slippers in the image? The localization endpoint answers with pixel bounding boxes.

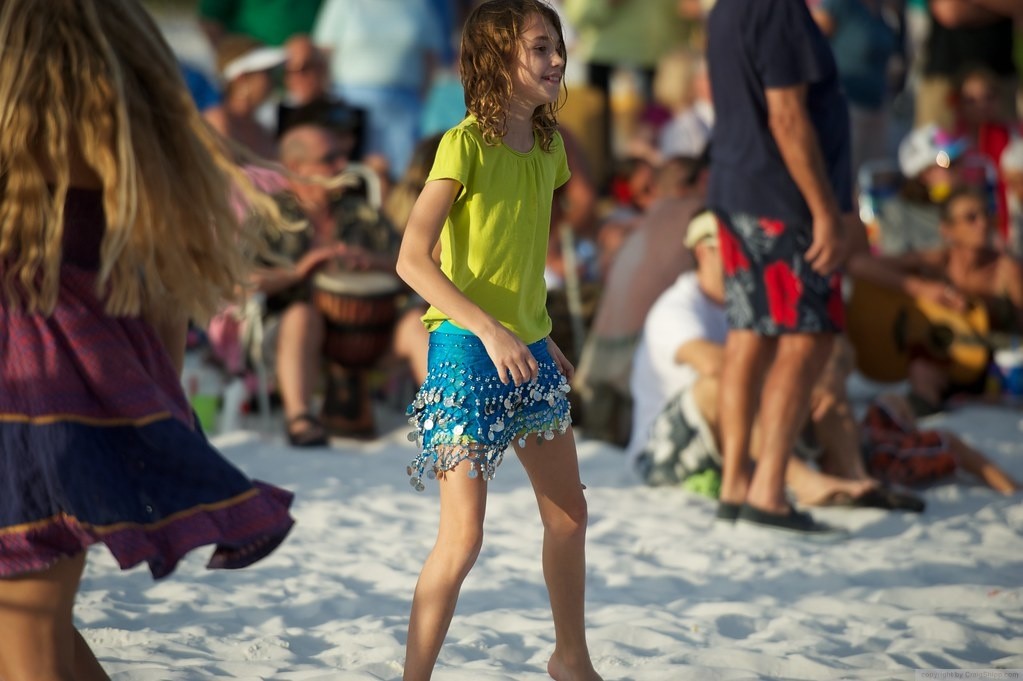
[716,503,745,520]
[736,499,816,532]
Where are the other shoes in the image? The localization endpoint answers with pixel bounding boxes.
[907,390,951,416]
[245,388,280,412]
[285,414,327,446]
[854,475,925,512]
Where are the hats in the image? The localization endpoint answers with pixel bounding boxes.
[897,123,969,180]
[683,210,719,250]
[223,45,291,81]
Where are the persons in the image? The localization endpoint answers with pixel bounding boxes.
[392,0,607,680]
[161,1,458,453]
[543,0,1023,531]
[0,0,360,681]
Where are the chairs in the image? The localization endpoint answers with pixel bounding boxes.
[231,161,382,423]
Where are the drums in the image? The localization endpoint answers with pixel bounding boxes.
[313,269,403,440]
